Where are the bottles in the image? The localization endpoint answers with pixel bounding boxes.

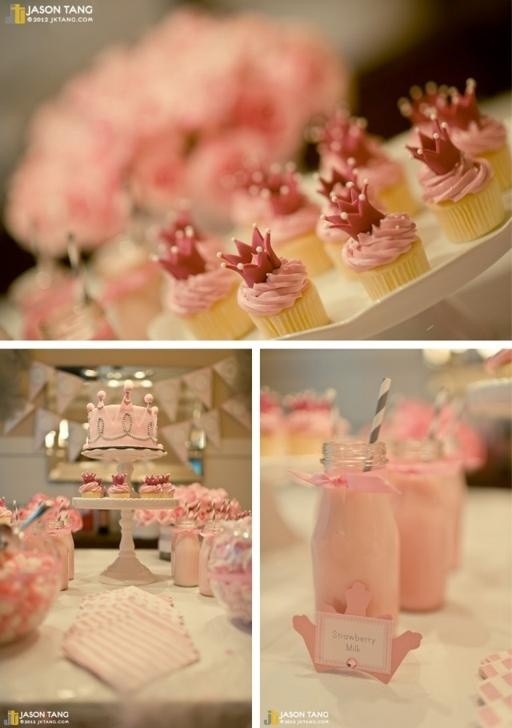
[0,518,74,648]
[371,433,467,617]
[311,441,402,645]
[168,510,252,627]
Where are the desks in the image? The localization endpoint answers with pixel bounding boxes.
[1,92,512,341]
[0,548,252,728]
[260,486,512,728]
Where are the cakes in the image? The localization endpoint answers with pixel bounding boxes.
[148,79,512,340]
[78,383,176,499]
